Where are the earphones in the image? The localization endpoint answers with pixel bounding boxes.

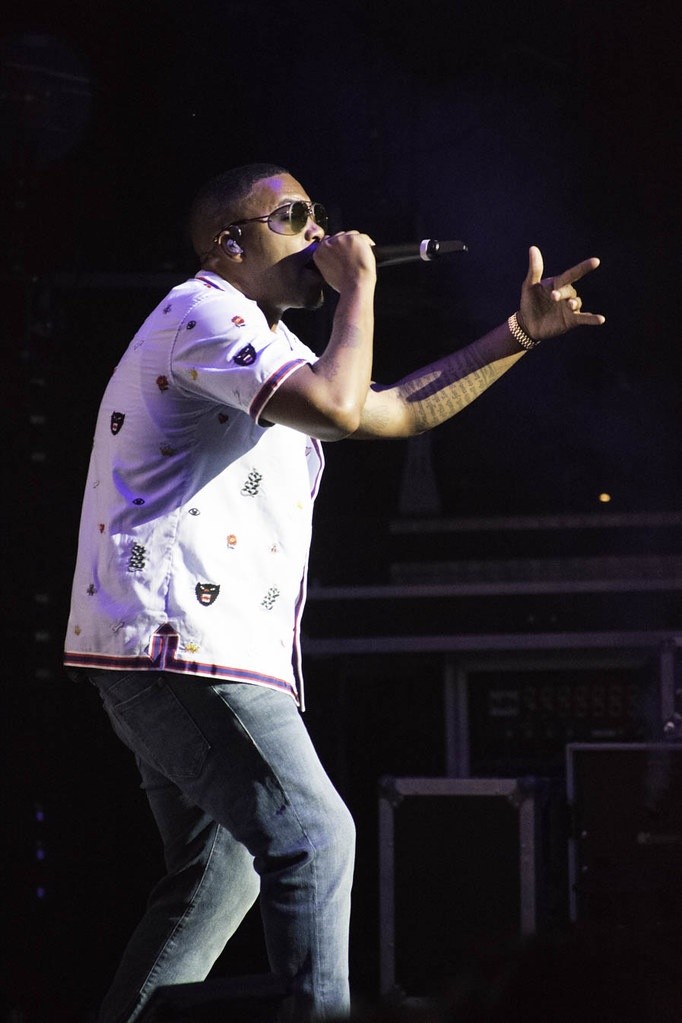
[227,239,243,255]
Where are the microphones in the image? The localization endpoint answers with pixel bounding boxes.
[371,238,469,267]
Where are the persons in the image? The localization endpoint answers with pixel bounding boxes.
[62,153,606,1023]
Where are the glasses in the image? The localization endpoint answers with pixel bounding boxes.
[213,201,329,243]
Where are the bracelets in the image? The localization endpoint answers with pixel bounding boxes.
[508,311,541,350]
